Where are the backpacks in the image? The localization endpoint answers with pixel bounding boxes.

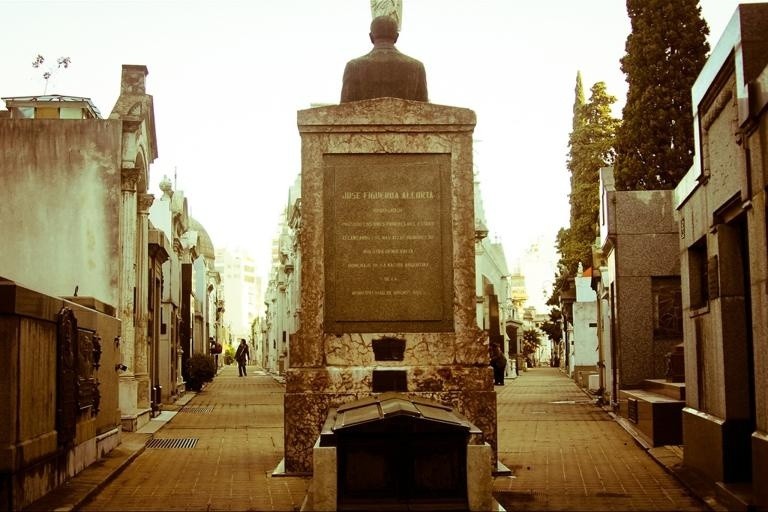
[215,342,222,353]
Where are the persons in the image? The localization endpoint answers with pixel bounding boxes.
[339,15,429,104]
[233,338,250,377]
[207,337,220,377]
[489,342,507,386]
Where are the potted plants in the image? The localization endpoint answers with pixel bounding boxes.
[184,353,218,391]
